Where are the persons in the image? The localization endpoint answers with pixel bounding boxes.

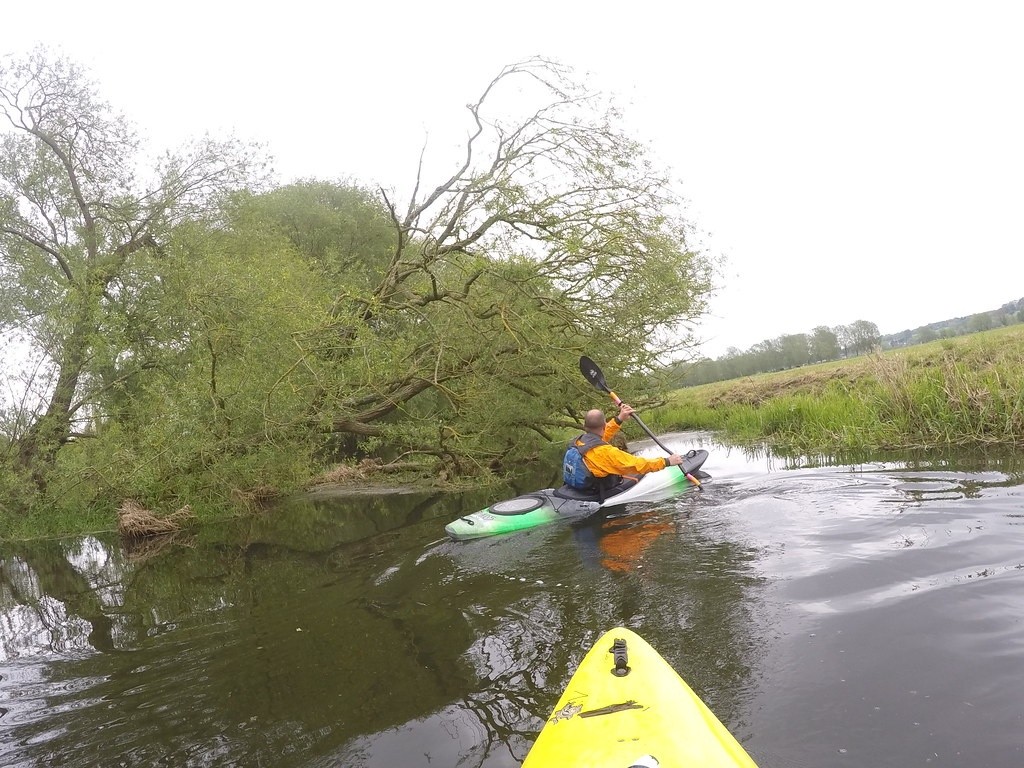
[568,404,684,493]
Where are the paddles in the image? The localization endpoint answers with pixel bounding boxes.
[577,352,706,491]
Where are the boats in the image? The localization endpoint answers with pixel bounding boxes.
[445,449,709,541]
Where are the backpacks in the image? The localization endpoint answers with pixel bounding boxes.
[563,433,609,504]
